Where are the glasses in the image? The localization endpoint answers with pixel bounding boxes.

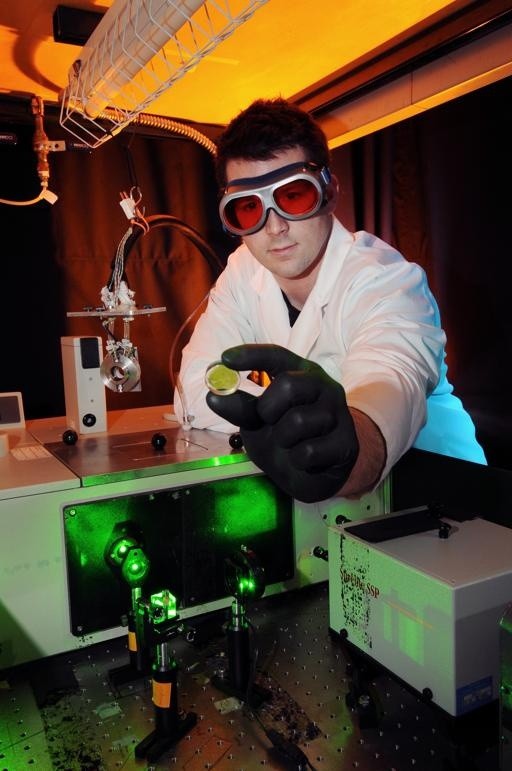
[215,162,333,234]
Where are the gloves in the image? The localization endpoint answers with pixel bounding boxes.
[204,344,358,503]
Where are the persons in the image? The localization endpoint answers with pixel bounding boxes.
[171,97,489,508]
